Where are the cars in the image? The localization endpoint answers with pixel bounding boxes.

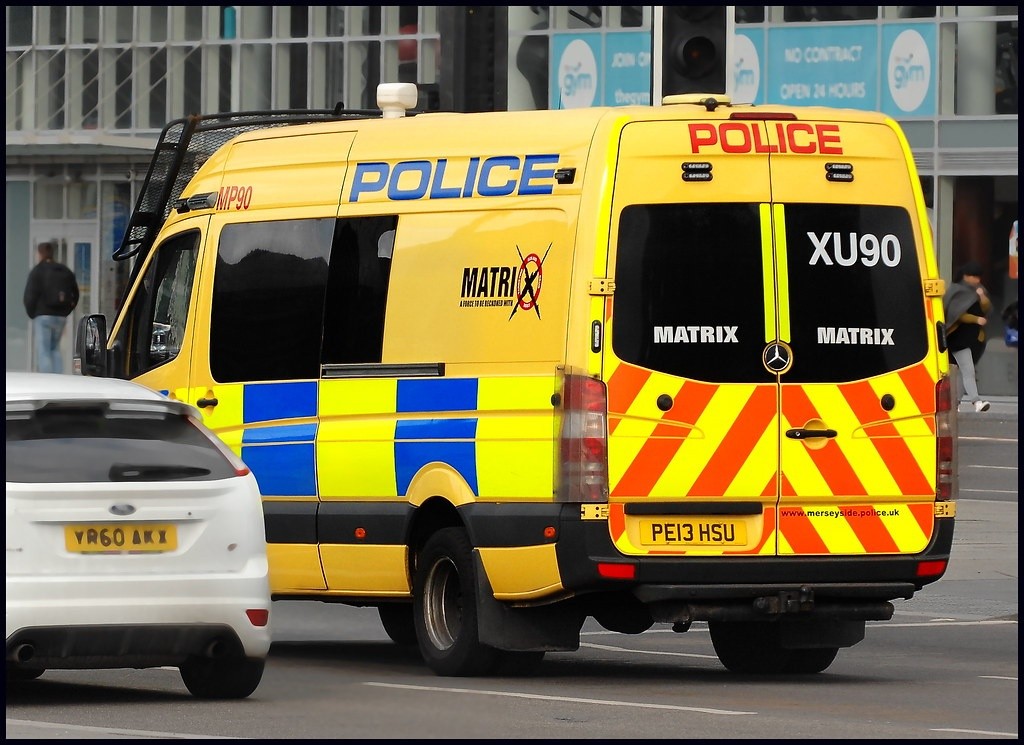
[7,370,273,702]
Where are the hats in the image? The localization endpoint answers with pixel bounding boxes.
[960,261,982,276]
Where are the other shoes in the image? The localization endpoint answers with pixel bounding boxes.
[975,400,991,413]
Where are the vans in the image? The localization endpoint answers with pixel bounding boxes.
[76,81,955,680]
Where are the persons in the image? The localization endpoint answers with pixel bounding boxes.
[23,243,80,375]
[942,262,992,411]
